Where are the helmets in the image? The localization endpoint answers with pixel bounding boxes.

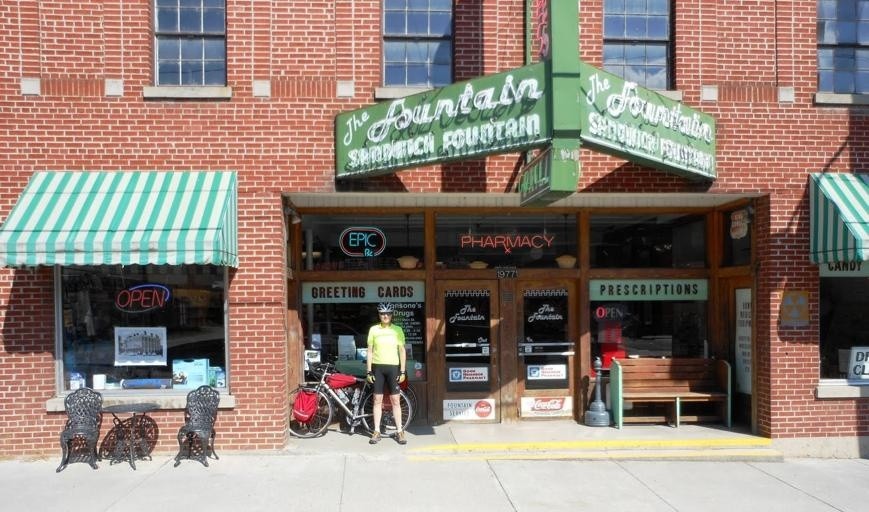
[377,302,394,312]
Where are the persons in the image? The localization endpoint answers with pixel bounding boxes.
[364,301,408,445]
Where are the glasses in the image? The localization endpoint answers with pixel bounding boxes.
[381,312,391,315]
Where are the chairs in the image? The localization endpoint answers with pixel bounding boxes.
[55,387,103,473]
[172,385,219,468]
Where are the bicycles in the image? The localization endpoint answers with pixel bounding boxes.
[288,357,417,438]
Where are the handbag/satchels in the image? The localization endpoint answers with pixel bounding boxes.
[293,390,318,423]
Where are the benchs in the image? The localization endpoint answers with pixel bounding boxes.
[609,358,732,429]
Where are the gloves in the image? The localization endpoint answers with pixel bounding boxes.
[366,371,376,384]
[397,373,406,384]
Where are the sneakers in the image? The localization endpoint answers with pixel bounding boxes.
[394,432,407,444]
[369,432,381,444]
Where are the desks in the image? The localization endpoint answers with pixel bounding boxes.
[100,402,161,471]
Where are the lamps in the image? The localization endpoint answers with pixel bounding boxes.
[396,215,419,269]
[555,215,576,269]
[284,205,302,224]
[470,223,489,270]
